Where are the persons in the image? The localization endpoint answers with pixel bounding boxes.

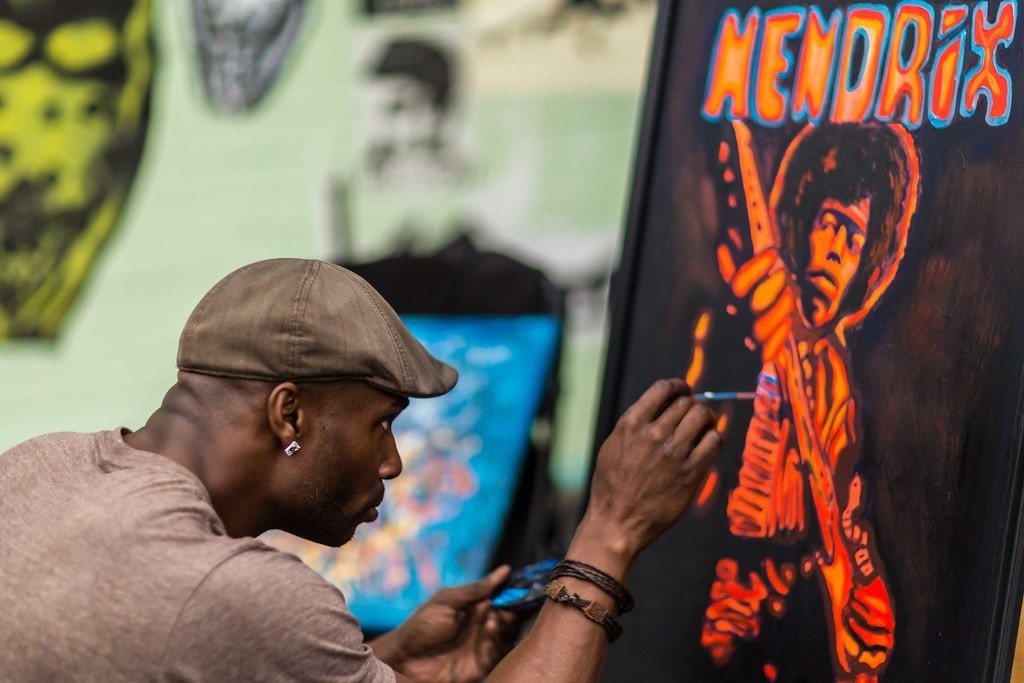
[2,257,732,682]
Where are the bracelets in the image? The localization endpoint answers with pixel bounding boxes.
[541,578,623,644]
[550,554,636,615]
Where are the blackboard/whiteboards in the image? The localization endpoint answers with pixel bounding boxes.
[556,0,1023,683]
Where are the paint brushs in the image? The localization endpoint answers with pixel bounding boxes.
[691,392,761,401]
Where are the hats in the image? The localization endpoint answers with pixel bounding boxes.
[177,259,460,397]
